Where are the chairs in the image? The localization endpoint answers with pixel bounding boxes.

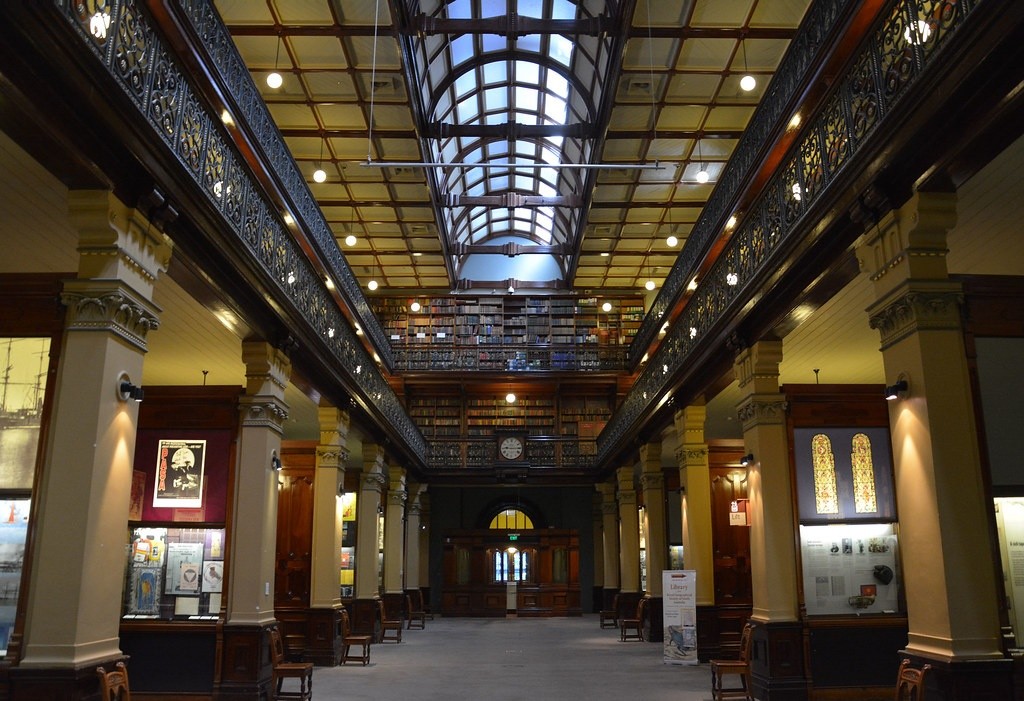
[338,609,373,667]
[599,594,621,629]
[266,625,314,701]
[378,600,403,644]
[894,658,936,701]
[708,623,758,701]
[420,590,434,620]
[619,598,648,642]
[405,595,426,630]
[96,661,132,701]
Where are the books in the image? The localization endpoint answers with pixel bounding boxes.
[598,299,644,371]
[526,400,553,457]
[409,399,461,457]
[454,298,502,370]
[503,300,550,370]
[369,298,407,370]
[561,408,610,456]
[467,400,524,458]
[407,297,454,370]
[550,298,598,371]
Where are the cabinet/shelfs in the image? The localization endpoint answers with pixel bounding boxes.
[364,294,647,371]
[407,392,615,466]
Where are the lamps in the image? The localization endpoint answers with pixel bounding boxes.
[120,381,145,403]
[615,516,622,521]
[740,453,753,467]
[637,504,646,510]
[345,207,357,247]
[340,488,346,496]
[265,35,283,89]
[505,384,516,404]
[885,380,908,401]
[675,486,685,495]
[666,208,677,248]
[739,38,757,91]
[645,256,655,291]
[507,285,515,295]
[600,524,604,528]
[601,287,612,312]
[419,525,426,530]
[312,138,326,183]
[696,138,709,184]
[378,507,384,514]
[402,518,408,522]
[273,456,283,471]
[367,256,378,291]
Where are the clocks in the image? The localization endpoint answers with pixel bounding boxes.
[493,429,529,462]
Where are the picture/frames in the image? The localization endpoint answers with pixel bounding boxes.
[152,440,207,508]
[128,567,163,615]
